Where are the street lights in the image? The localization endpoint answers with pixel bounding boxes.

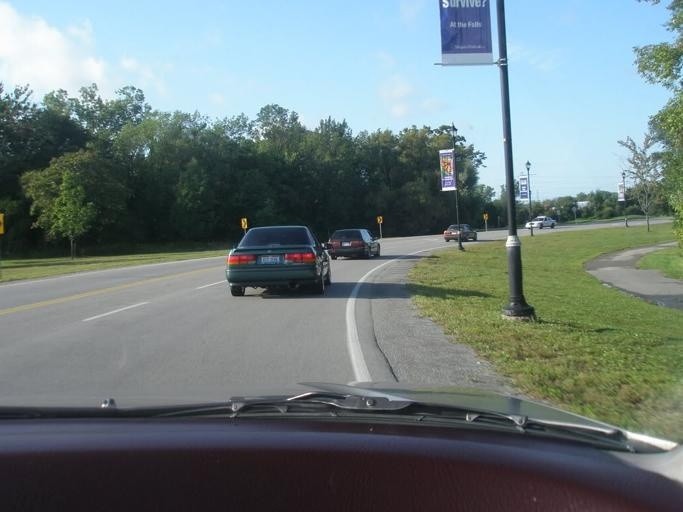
[449,123,464,251]
[622,171,628,227]
[526,160,533,236]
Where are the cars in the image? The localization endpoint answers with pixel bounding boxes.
[225,226,331,297]
[526,216,557,229]
[444,224,477,242]
[327,229,380,259]
